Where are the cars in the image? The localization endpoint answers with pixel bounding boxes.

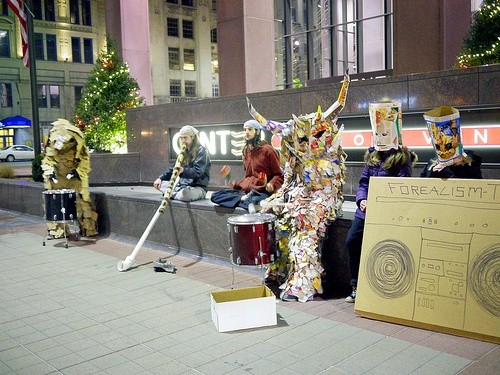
[0,143,40,163]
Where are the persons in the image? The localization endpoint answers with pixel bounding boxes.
[31,141,51,182]
[420,105,484,180]
[242,68,351,306]
[154,126,212,204]
[41,119,97,240]
[210,118,287,213]
[344,100,418,304]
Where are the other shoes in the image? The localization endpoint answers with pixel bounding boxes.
[345,287,356,303]
[247,189,261,198]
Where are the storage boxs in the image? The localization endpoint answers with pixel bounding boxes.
[209,285,278,333]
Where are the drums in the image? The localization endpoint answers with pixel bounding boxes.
[227,212,277,267]
[42,189,77,224]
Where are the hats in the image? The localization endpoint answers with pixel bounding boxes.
[243,119,263,129]
[180,125,198,136]
